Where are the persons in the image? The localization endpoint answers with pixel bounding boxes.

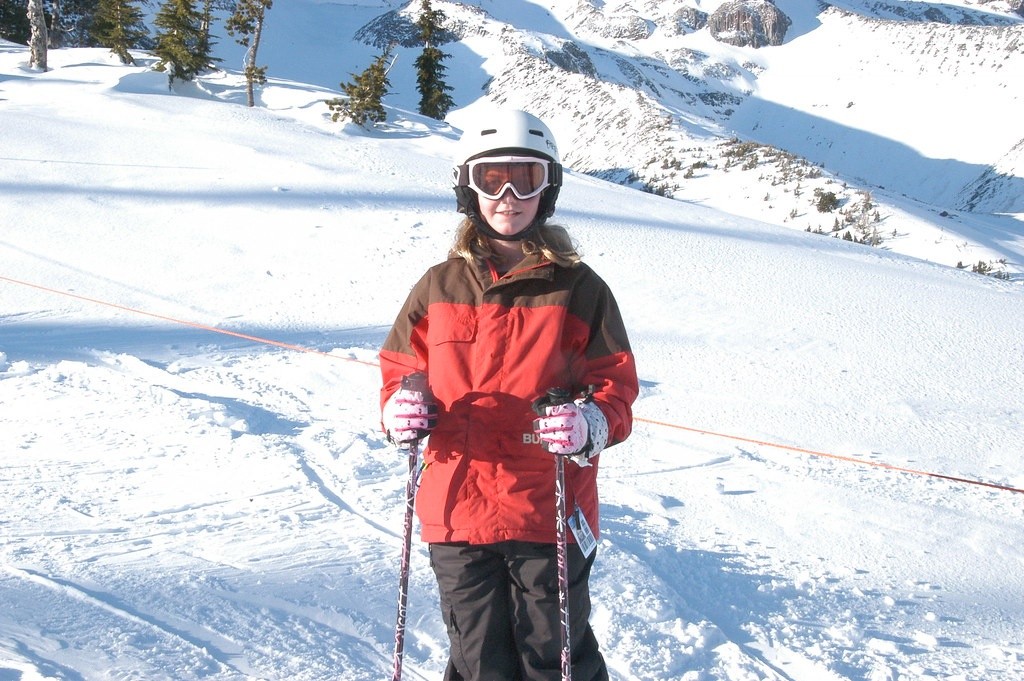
[380,110,639,681]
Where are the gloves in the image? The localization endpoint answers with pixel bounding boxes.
[381,375,439,451]
[531,393,609,467]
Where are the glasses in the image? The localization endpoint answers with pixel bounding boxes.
[451,155,562,201]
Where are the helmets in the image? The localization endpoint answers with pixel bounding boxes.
[449,109,560,189]
